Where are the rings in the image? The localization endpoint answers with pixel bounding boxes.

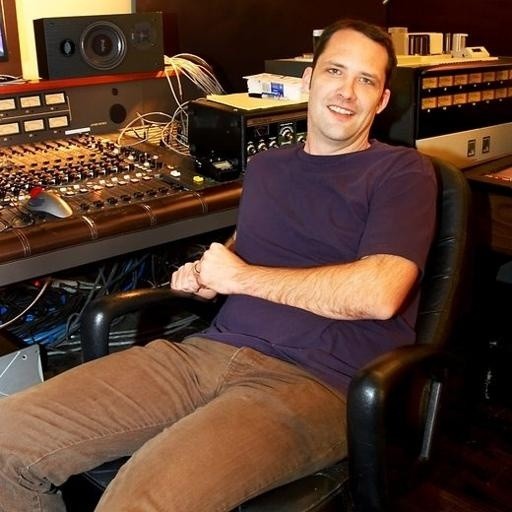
[194,264,200,274]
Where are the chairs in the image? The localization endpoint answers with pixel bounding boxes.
[70,141,493,503]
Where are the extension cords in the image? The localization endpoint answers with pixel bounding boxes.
[38,278,79,293]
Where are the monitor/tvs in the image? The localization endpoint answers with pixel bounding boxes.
[0,0,10,62]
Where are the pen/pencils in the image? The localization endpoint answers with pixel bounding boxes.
[248,92,280,99]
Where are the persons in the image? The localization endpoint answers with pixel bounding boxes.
[0,12,440,511]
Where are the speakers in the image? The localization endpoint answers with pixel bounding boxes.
[33,11,165,81]
